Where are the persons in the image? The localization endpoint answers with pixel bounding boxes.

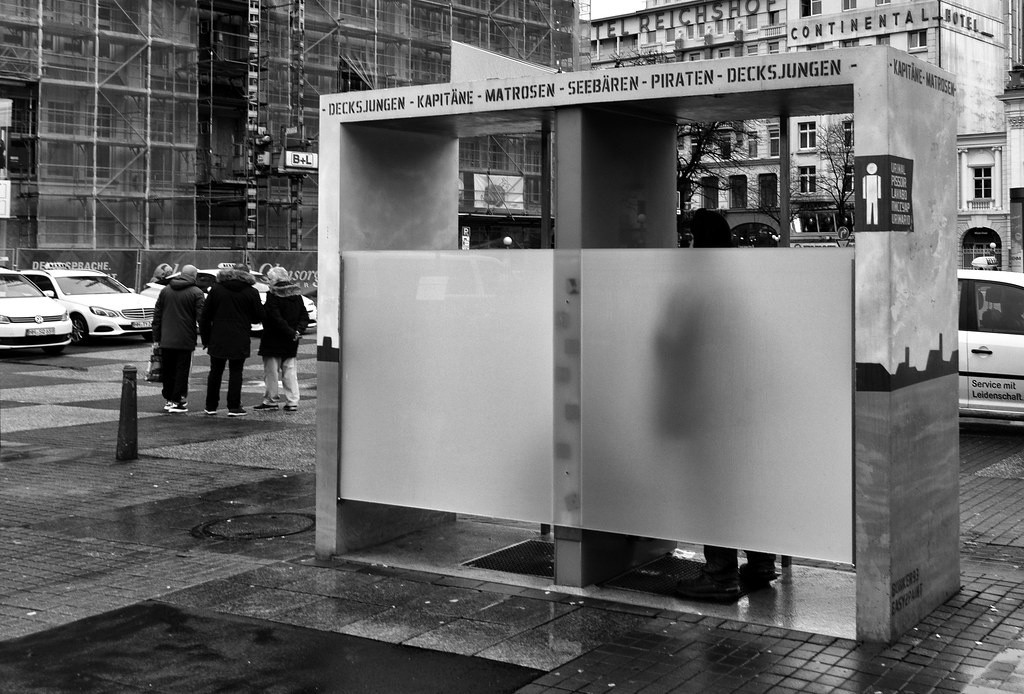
[152,264,205,413]
[199,263,261,417]
[251,266,311,411]
[150,264,173,284]
[649,207,775,599]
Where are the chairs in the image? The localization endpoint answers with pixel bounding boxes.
[981,287,1002,328]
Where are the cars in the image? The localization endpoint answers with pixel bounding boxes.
[139,263,317,331]
[0,268,73,354]
[958,269,1024,426]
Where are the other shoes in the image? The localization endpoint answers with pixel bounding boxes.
[740,563,776,579]
[284,405,298,411]
[253,404,279,410]
[168,402,188,412]
[675,576,738,597]
[227,405,247,416]
[164,401,188,410]
[204,408,217,414]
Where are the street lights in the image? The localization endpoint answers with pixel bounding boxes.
[503,236,512,249]
[638,214,645,241]
[989,243,996,257]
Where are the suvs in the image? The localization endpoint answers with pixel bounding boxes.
[18,269,158,346]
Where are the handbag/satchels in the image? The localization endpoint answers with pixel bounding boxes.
[145,347,162,383]
